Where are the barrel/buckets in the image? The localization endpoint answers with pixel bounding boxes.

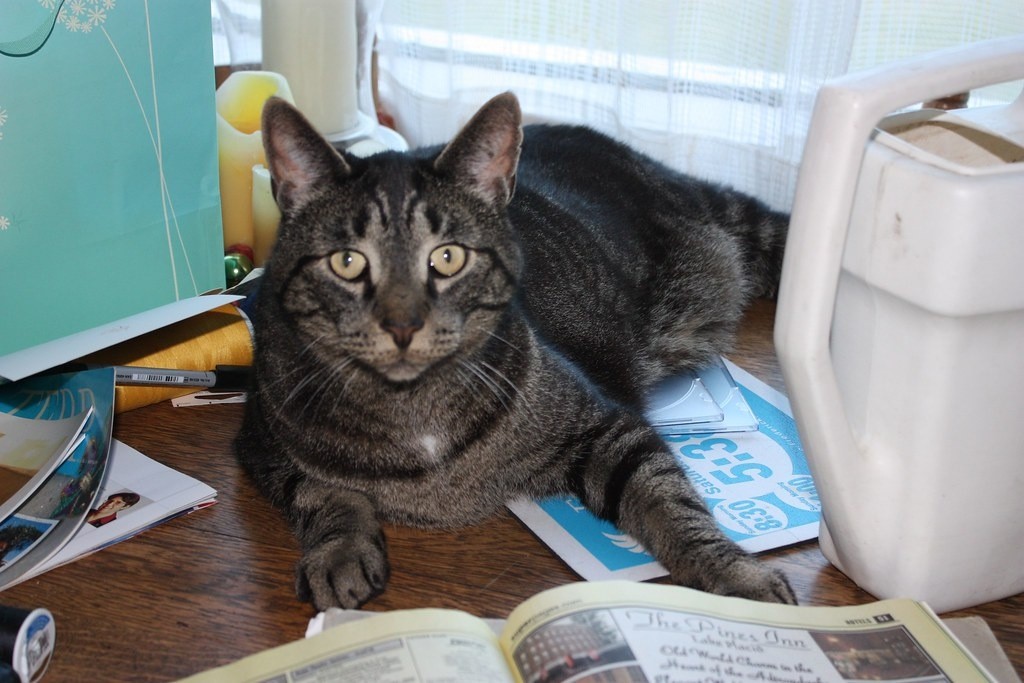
[772,42,1024,615]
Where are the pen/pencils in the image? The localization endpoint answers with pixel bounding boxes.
[106,364,254,391]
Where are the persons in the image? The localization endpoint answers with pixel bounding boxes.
[85,492,140,529]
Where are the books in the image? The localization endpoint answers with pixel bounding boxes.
[167,577,1020,683]
[0,367,220,595]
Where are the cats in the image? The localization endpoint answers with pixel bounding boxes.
[230,91,799,613]
